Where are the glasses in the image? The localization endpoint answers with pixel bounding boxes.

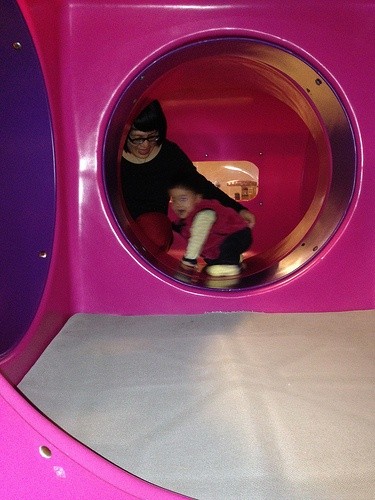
[128,134,160,145]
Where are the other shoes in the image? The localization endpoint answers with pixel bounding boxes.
[206,265,241,277]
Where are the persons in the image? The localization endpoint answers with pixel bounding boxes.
[166,173,252,279]
[115,92,256,256]
